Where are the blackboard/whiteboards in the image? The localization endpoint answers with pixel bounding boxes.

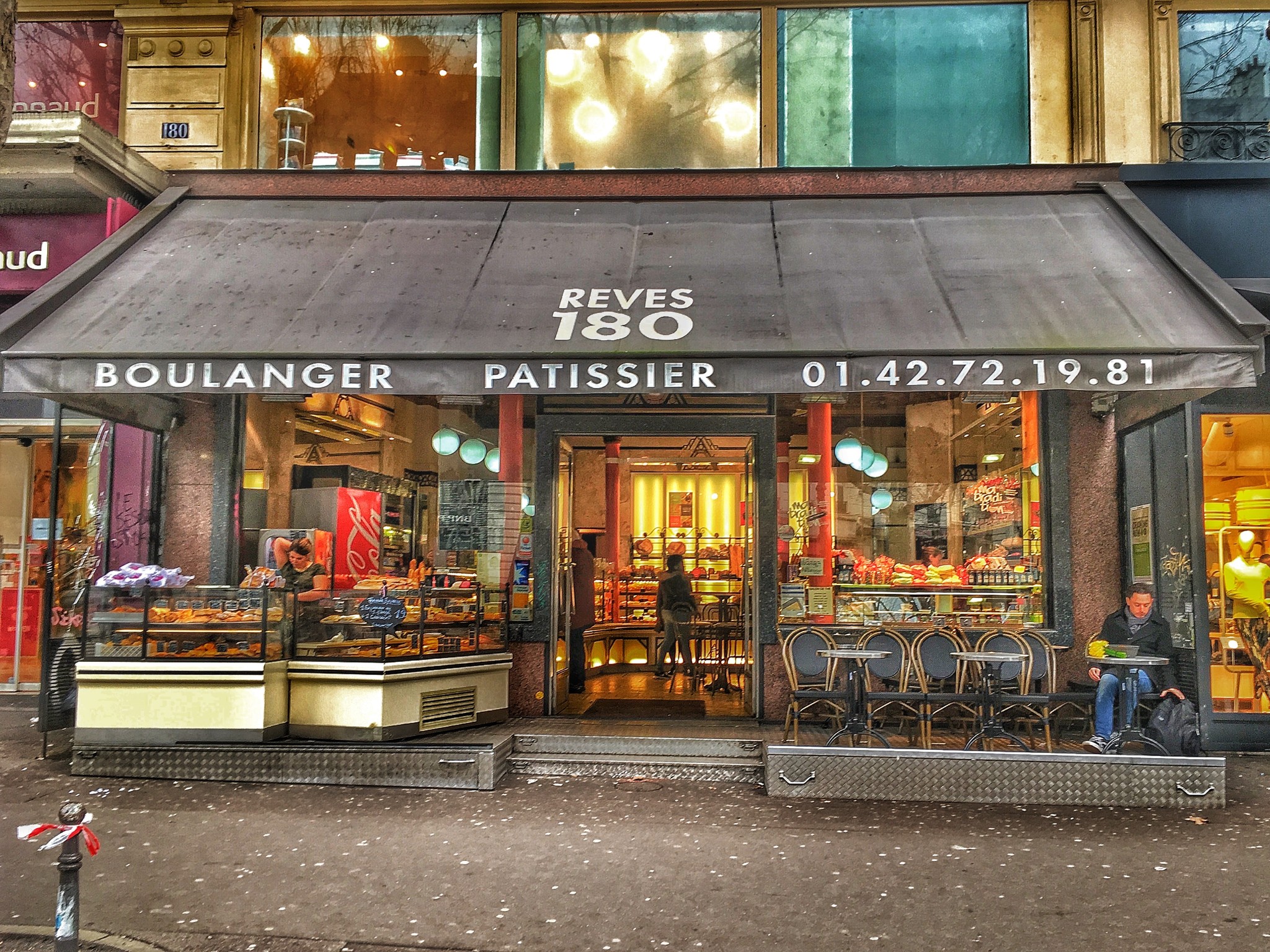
[437,479,502,551]
[357,595,408,638]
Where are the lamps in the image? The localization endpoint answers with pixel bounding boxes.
[428,425,501,474]
[521,493,535,518]
[1235,484,1270,526]
[836,434,889,478]
[870,488,894,510]
[1201,499,1232,536]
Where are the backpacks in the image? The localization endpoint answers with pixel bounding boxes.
[1144,695,1206,757]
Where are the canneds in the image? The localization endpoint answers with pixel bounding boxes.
[967,569,1034,583]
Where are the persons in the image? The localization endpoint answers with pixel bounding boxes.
[921,546,943,567]
[1083,582,1185,754]
[1259,554,1270,599]
[1224,530,1270,712]
[424,551,433,568]
[568,528,595,693]
[653,554,708,680]
[274,538,329,641]
[1207,563,1220,599]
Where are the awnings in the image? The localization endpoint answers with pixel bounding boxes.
[0,181,1270,436]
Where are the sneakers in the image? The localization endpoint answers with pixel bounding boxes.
[1082,734,1107,753]
[686,672,706,680]
[653,671,671,679]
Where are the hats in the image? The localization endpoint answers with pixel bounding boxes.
[572,528,581,542]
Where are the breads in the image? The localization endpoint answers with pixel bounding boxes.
[355,559,430,590]
[239,567,273,588]
[321,605,503,656]
[105,607,285,660]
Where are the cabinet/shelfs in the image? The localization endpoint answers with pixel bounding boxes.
[75,568,292,659]
[592,533,757,624]
[295,577,513,661]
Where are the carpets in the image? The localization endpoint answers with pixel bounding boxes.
[581,698,707,719]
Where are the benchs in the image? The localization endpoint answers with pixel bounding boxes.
[1081,655,1171,756]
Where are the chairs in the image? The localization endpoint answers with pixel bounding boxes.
[669,601,753,695]
[775,621,849,733]
[977,630,1054,754]
[882,625,956,734]
[1008,629,1102,749]
[853,627,927,749]
[1084,625,1169,701]
[953,626,1022,734]
[779,623,856,746]
[908,629,988,750]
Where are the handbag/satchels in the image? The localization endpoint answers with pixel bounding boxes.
[671,599,694,621]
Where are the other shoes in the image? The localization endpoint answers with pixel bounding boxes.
[569,685,586,694]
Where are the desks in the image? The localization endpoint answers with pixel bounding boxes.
[823,641,879,728]
[949,651,1030,753]
[683,589,742,694]
[812,647,896,748]
[1017,641,1072,732]
